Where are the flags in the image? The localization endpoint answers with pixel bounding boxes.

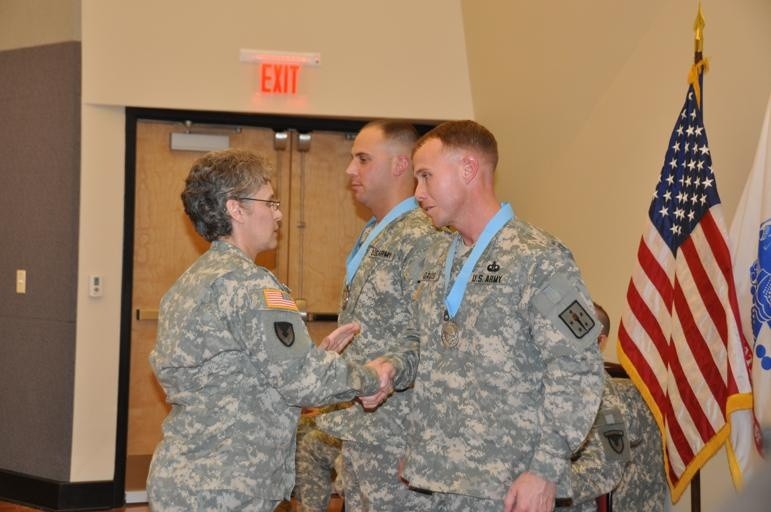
[616,5,758,504]
[729,97,771,463]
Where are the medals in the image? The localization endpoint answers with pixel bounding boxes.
[340,286,350,310]
[442,320,458,348]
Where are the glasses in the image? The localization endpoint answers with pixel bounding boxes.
[237,197,281,210]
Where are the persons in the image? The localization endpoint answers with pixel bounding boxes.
[353,117,609,510]
[561,301,677,511]
[146,144,400,512]
[289,114,452,510]
